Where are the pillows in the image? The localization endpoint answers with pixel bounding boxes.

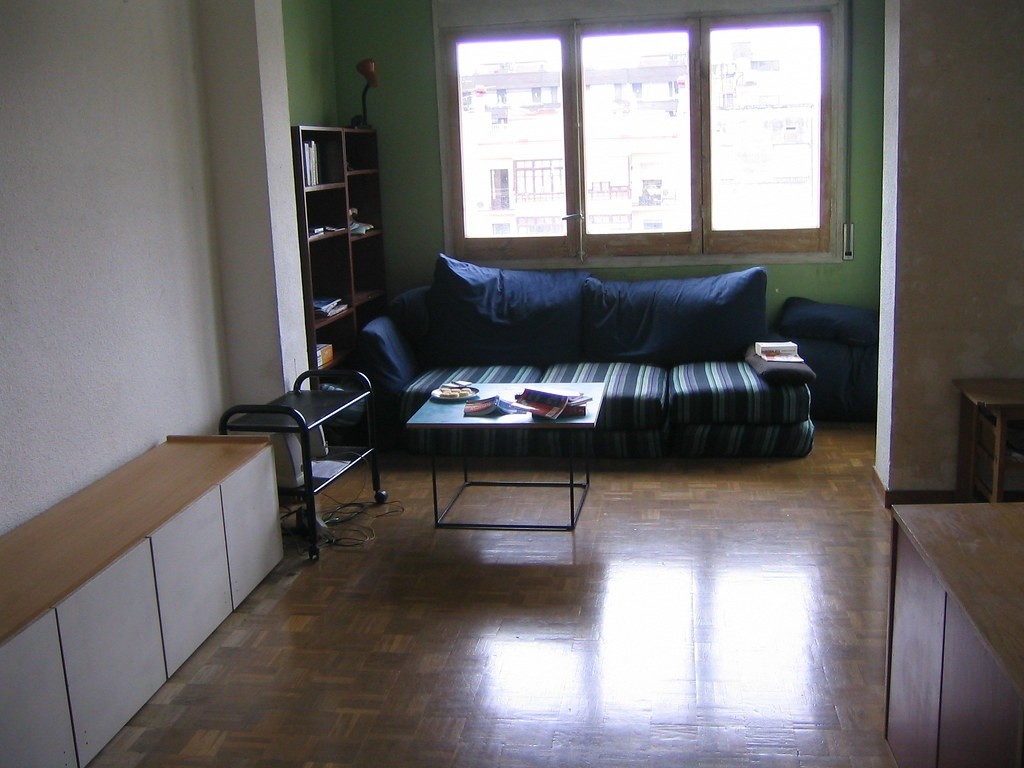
[421,252,589,363]
[777,297,879,346]
[582,266,768,362]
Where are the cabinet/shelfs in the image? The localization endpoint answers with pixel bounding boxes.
[0,427,284,768]
[219,370,389,560]
[293,124,397,449]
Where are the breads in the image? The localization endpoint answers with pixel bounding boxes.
[439,387,472,398]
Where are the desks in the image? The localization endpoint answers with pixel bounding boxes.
[405,380,608,530]
[882,502,1024,768]
[952,378,1024,502]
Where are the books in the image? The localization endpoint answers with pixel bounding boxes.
[302,141,318,186]
[308,225,324,239]
[464,395,526,416]
[511,387,593,420]
[313,298,348,316]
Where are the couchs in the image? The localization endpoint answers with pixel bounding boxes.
[360,284,815,458]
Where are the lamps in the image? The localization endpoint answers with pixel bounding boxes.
[350,57,379,128]
